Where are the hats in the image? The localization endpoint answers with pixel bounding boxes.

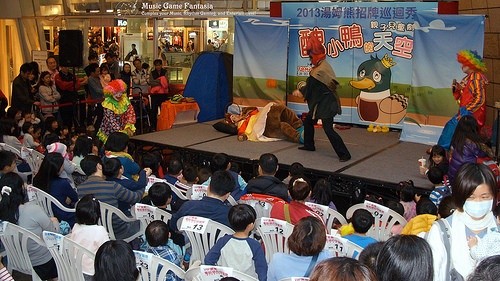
[46,142,67,157]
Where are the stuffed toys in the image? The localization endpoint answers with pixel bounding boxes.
[212,102,304,145]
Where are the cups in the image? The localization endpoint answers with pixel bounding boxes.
[417,158,427,175]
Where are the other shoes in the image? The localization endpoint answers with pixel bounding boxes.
[298,146,315,151]
[339,155,351,162]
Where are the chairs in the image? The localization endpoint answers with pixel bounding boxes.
[0,144,409,281]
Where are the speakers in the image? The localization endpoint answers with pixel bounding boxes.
[58,30,83,67]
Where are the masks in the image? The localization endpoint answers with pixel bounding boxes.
[462,200,493,219]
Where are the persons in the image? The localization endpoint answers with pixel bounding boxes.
[448,115,494,187]
[426,161,500,281]
[420,144,453,194]
[207,36,221,52]
[95,132,182,281]
[0,106,97,230]
[427,167,453,217]
[0,172,70,280]
[0,35,171,136]
[307,174,457,281]
[60,194,110,281]
[204,204,268,281]
[297,36,351,162]
[266,216,333,281]
[139,220,183,281]
[176,153,313,281]
[423,49,492,157]
[96,79,137,145]
